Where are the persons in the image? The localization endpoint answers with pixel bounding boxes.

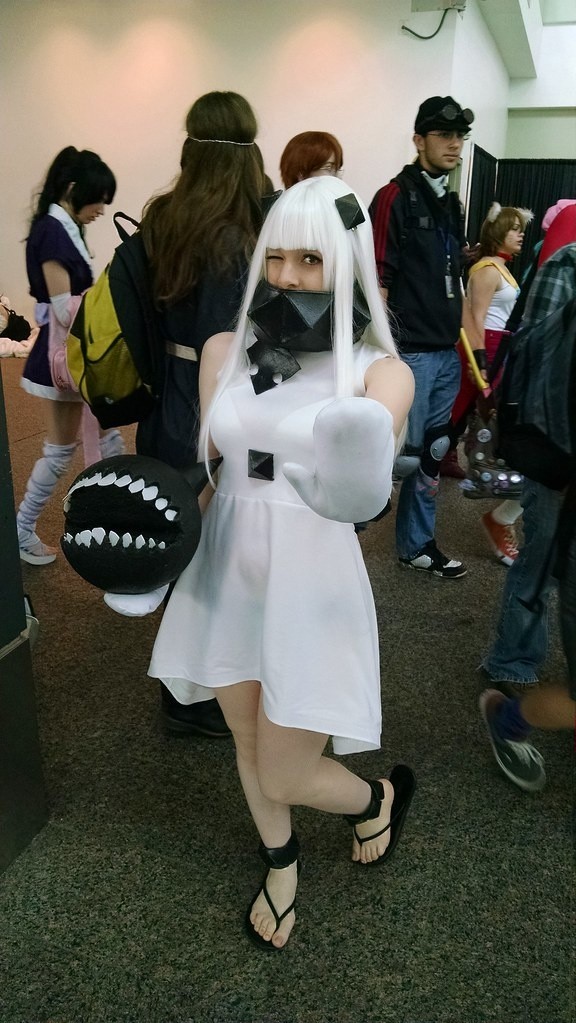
[480,678,576,790]
[443,200,574,562]
[105,176,415,953]
[370,97,467,577]
[14,145,122,565]
[279,131,343,189]
[66,93,274,733]
[479,241,575,699]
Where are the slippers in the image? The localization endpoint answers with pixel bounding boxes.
[345,765,417,867]
[245,830,301,952]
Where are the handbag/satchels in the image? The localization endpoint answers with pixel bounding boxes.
[461,397,526,500]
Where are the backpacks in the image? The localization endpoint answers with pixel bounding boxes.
[65,212,157,428]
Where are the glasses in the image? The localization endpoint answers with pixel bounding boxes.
[318,166,341,178]
[426,108,474,124]
[427,130,470,141]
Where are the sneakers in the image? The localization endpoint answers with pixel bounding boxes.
[398,541,468,579]
[479,690,548,788]
[477,510,522,566]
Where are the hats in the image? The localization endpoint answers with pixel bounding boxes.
[414,96,472,135]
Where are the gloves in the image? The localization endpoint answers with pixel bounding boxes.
[282,398,393,524]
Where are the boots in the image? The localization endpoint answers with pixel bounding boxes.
[96,429,125,458]
[438,449,465,480]
[18,440,77,565]
